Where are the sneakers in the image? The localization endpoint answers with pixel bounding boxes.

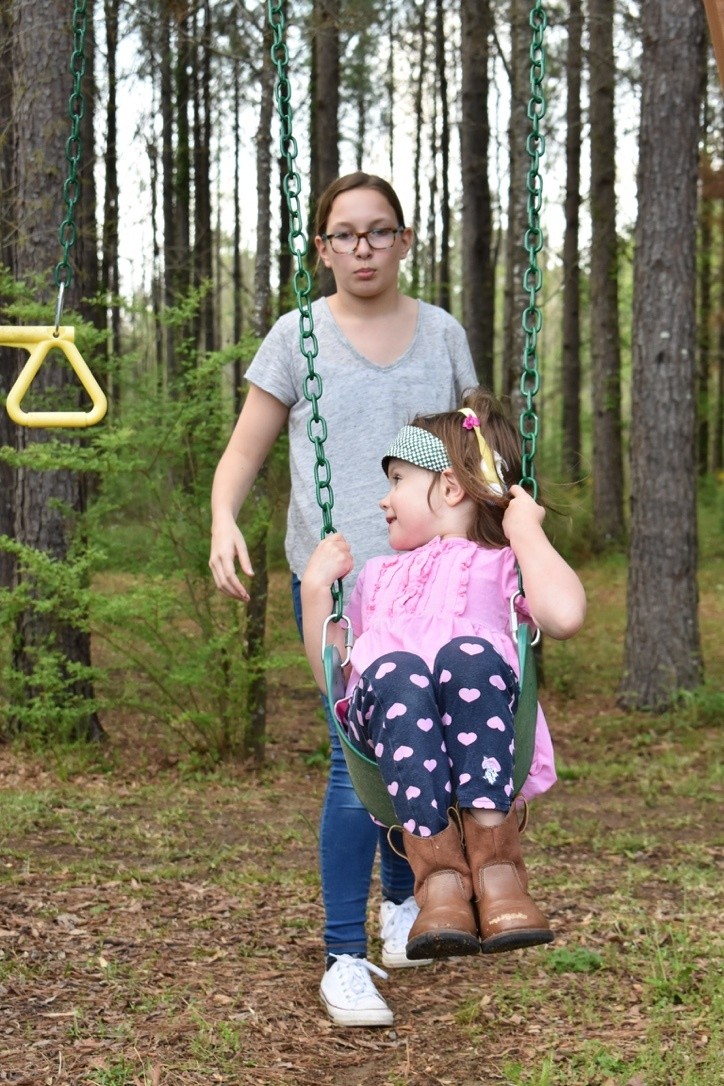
[318,952,394,1027]
[379,896,433,969]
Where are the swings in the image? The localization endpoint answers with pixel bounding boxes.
[260,0,555,826]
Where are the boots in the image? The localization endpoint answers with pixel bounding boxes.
[453,792,554,954]
[387,806,480,960]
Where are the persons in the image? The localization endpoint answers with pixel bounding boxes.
[210,174,485,1028]
[305,397,586,957]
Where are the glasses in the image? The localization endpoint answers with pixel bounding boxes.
[322,225,403,255]
